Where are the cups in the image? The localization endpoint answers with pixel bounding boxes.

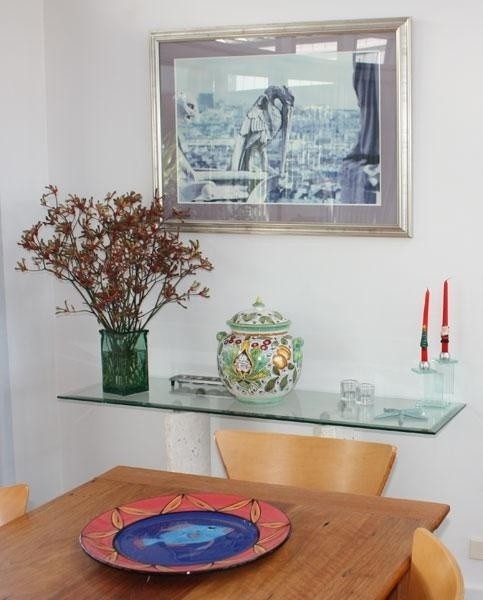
[339,378,358,401]
[355,383,375,405]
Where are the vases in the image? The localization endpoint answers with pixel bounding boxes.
[99,329,148,398]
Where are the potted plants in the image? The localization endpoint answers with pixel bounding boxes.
[16,186,213,390]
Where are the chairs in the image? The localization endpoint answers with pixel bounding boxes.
[216,431,396,498]
[0,484,28,529]
[406,527,465,600]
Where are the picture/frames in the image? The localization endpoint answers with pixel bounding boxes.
[150,18,413,239]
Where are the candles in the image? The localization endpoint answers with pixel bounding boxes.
[421,289,429,361]
[440,278,451,352]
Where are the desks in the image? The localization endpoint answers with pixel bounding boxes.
[0,465,450,600]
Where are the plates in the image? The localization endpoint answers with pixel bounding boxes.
[78,493,290,574]
